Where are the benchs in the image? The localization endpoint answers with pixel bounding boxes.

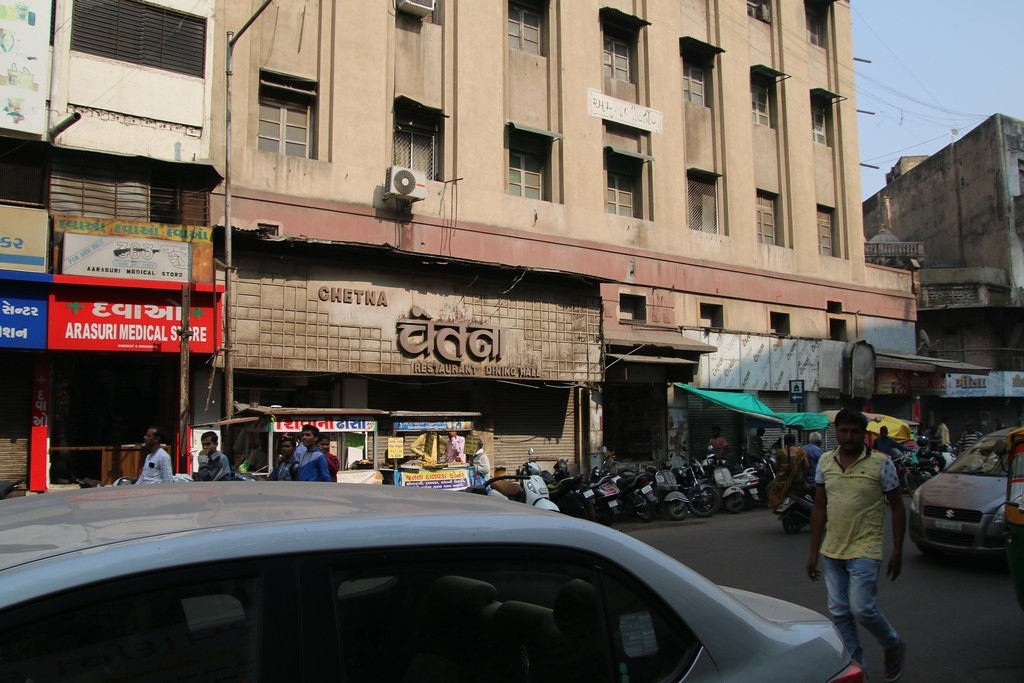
[434,576,594,683]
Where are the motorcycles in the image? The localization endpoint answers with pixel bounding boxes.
[1004,425,1024,615]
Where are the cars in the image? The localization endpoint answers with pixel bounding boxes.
[0,478,871,683]
[907,425,1015,574]
[891,431,956,496]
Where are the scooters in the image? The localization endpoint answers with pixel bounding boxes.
[458,440,780,527]
[774,484,825,535]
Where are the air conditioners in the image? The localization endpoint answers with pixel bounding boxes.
[385,166,428,201]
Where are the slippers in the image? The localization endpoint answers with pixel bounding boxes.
[885,640,906,682]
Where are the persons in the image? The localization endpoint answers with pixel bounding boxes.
[473,439,491,481]
[955,421,983,453]
[267,424,340,483]
[446,430,466,463]
[807,406,907,683]
[410,431,448,462]
[801,432,825,483]
[198,431,232,482]
[134,427,174,485]
[936,418,952,445]
[247,431,269,471]
[872,425,914,455]
[747,427,766,458]
[707,426,729,460]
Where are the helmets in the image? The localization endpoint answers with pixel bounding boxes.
[917,435,927,446]
